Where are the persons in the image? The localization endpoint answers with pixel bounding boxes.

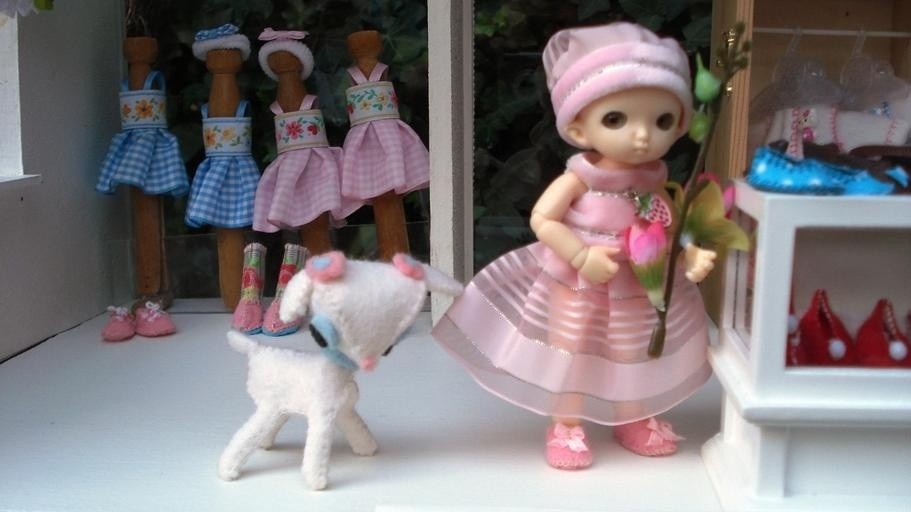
[427,17,716,468]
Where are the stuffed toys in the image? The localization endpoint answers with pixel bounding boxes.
[216,250,429,489]
